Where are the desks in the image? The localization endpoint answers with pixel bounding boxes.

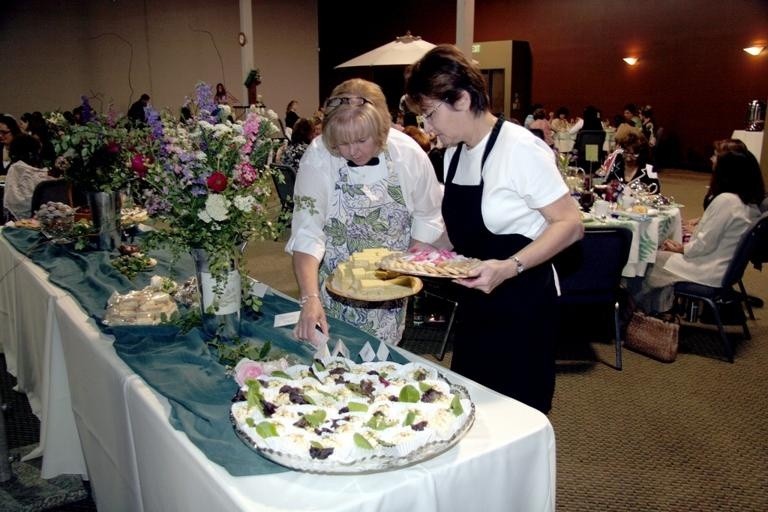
[0,220,558,512]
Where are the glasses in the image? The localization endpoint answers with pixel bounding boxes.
[0,130,10,135]
[324,96,377,109]
[622,151,640,158]
[420,97,450,123]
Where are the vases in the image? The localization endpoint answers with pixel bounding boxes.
[87,190,120,251]
[191,241,244,339]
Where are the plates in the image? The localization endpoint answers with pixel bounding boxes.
[375,249,482,279]
[324,268,423,302]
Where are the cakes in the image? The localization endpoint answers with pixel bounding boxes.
[380,244,482,277]
[325,247,421,299]
[102,289,177,329]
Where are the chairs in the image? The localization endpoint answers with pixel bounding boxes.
[669,213,768,364]
[530,127,545,141]
[31,179,70,217]
[577,130,607,173]
[269,163,298,242]
[556,226,635,372]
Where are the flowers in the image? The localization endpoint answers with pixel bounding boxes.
[122,82,314,247]
[50,99,151,187]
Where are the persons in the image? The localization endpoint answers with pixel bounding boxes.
[281,118,315,177]
[213,83,228,106]
[521,100,605,168]
[619,148,767,327]
[2,134,49,222]
[0,115,21,175]
[402,43,586,416]
[395,65,418,126]
[21,93,97,142]
[402,126,432,152]
[283,78,447,347]
[602,126,662,196]
[128,93,151,129]
[689,138,747,226]
[510,255,522,273]
[285,99,302,129]
[596,105,664,138]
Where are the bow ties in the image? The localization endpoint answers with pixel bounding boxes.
[347,157,380,168]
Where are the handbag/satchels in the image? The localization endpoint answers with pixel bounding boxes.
[624,311,681,363]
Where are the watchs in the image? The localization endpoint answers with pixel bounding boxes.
[298,293,321,306]
